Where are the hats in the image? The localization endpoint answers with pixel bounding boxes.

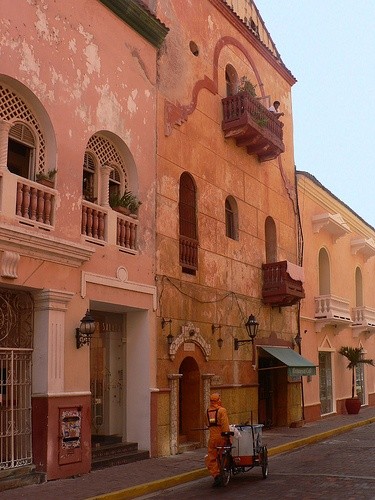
[209,393,221,405]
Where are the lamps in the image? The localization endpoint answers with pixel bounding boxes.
[234,314,260,351]
[76,309,96,349]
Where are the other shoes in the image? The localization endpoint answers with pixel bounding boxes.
[212,476,222,488]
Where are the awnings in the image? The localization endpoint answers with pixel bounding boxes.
[259,345,318,377]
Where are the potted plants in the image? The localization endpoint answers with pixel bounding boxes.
[109,190,140,220]
[338,345,375,415]
[36,169,57,188]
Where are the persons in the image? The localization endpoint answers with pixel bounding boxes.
[268,100,284,117]
[205,393,232,488]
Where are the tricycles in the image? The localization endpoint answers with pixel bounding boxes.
[214,410,269,487]
